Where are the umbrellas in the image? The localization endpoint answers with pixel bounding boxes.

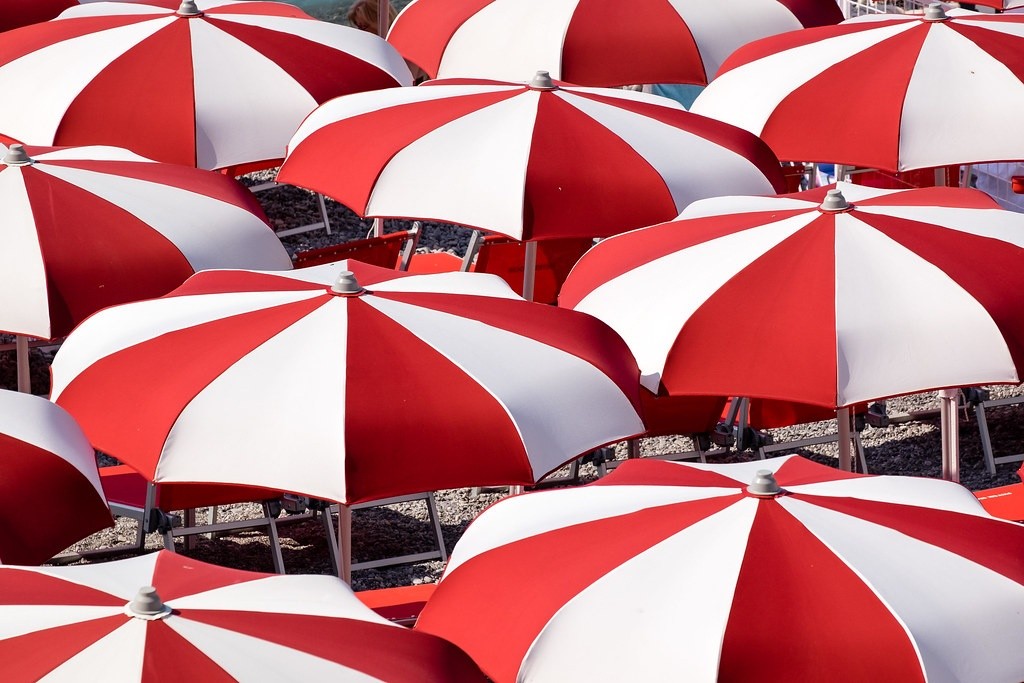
[0,0,1024,683]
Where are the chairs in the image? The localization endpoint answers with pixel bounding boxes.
[79,154,1024,633]
